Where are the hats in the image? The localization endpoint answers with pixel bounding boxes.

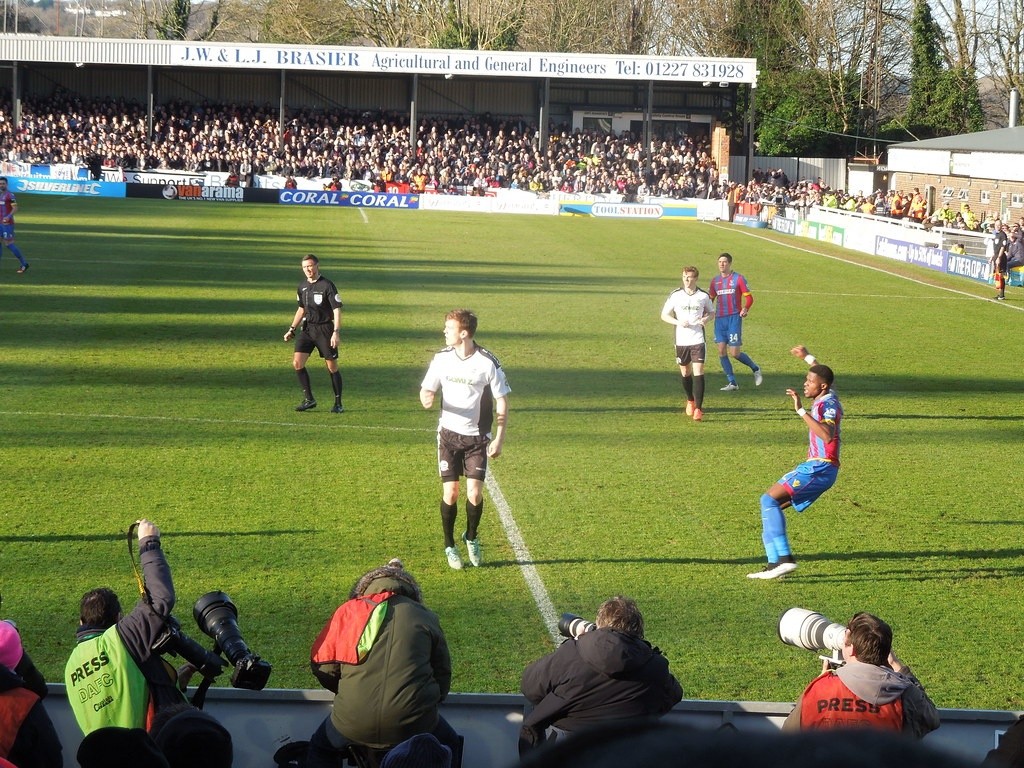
[77,728,169,768]
[942,200,950,205]
[956,211,962,218]
[156,710,233,768]
[0,621,22,670]
[380,733,452,768]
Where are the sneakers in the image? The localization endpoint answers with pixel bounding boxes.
[686,400,695,415]
[753,365,763,386]
[334,404,343,412]
[17,264,29,273]
[747,561,797,579]
[993,295,1005,301]
[721,382,739,390]
[462,532,482,567]
[694,409,701,421]
[445,545,464,569]
[296,398,317,411]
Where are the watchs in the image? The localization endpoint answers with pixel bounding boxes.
[289,325,297,331]
[333,329,340,332]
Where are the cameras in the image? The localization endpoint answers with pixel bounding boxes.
[777,607,846,652]
[192,590,271,691]
[557,614,597,640]
[149,614,230,684]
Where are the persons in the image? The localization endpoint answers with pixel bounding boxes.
[721,165,902,224]
[0,177,30,274]
[902,187,1024,300]
[747,344,844,581]
[516,713,1023,767]
[782,610,941,745]
[284,255,344,413]
[516,594,683,768]
[659,265,716,422]
[705,253,762,390]
[63,520,191,731]
[0,109,721,200]
[419,308,511,570]
[0,595,457,768]
[310,559,462,768]
